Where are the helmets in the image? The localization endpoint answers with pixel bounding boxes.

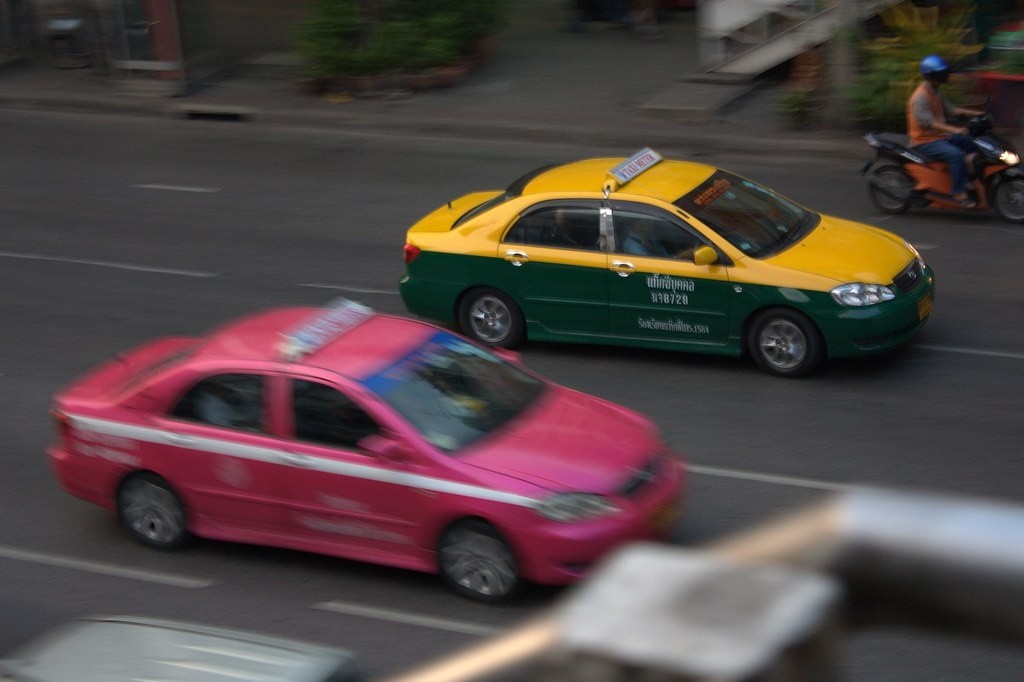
[921,55,950,74]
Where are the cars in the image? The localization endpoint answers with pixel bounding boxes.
[397,148,936,377]
[45,308,685,605]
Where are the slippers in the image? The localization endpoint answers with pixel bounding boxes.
[948,194,978,209]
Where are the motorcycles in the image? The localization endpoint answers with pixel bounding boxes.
[862,109,1024,223]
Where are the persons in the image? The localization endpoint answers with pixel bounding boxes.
[621,217,697,261]
[906,56,986,208]
[199,382,268,434]
